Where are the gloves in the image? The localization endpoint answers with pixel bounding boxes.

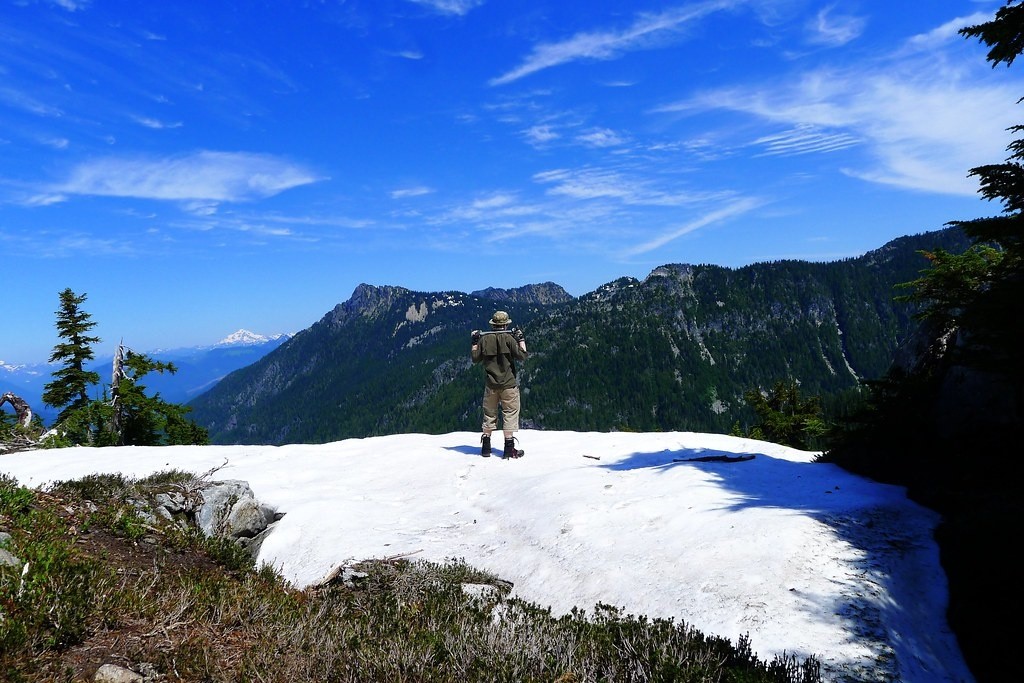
[511,325,525,343]
[471,330,482,346]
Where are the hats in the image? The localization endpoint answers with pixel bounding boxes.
[489,311,512,325]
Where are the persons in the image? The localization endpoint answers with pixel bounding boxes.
[471,311,524,459]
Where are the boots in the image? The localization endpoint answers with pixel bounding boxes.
[502,437,524,459]
[480,434,492,457]
